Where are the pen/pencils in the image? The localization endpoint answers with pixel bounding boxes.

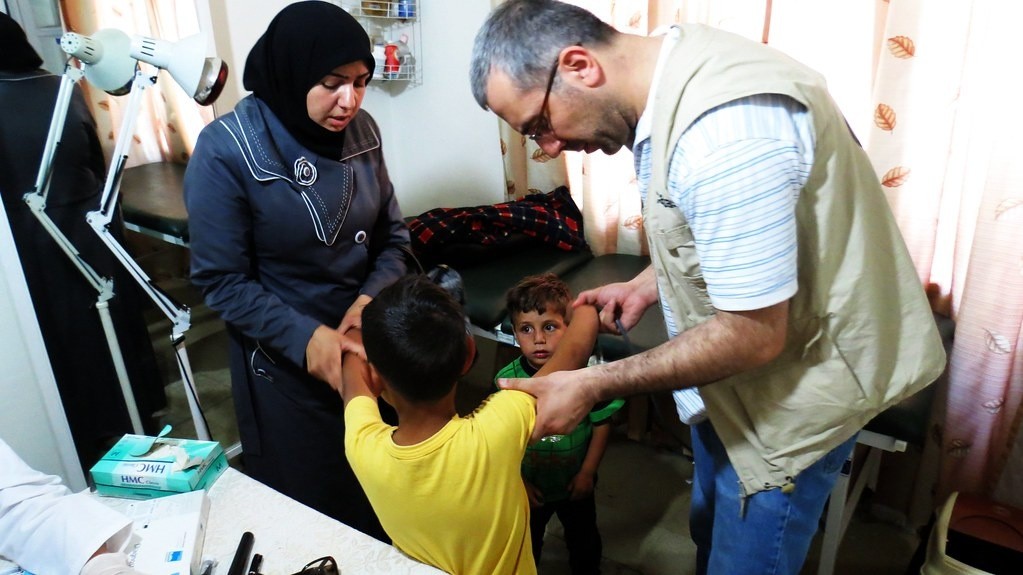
[248,554,262,575]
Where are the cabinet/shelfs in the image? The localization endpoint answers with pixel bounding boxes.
[349,0,424,83]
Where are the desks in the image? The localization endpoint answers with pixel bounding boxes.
[0,456,455,575]
[98,158,253,252]
[508,253,955,575]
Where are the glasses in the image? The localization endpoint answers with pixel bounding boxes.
[528,40,582,142]
[291,555,338,575]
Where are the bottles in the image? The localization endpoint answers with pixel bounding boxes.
[368,25,413,81]
[397,0,416,18]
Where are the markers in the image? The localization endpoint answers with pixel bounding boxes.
[228,532,253,575]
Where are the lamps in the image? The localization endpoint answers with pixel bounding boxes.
[59,26,228,106]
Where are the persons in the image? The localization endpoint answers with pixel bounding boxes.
[493,274,625,575]
[0,438,151,575]
[469,0,946,575]
[341,274,601,575]
[0,10,166,478]
[182,0,410,546]
